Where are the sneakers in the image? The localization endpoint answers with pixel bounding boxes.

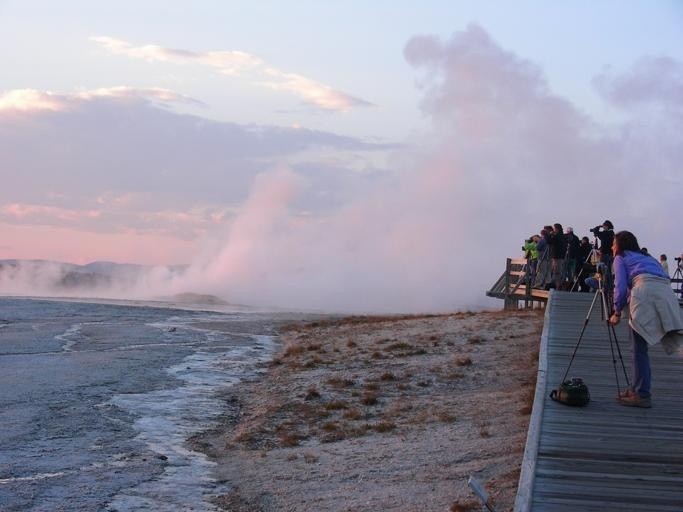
[616,388,651,408]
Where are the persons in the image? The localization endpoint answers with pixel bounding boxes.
[607,230,671,410]
[523,219,614,294]
[641,248,648,253]
[677,252,682,299]
[659,254,668,273]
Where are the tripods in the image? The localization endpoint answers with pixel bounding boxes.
[569,238,602,294]
[671,262,683,296]
[561,276,630,397]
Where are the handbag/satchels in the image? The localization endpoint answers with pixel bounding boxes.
[549,377,591,408]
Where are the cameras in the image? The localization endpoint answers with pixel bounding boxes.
[675,257,681,261]
[590,228,599,236]
[597,262,607,275]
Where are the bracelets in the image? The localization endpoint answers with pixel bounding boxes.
[613,313,621,317]
